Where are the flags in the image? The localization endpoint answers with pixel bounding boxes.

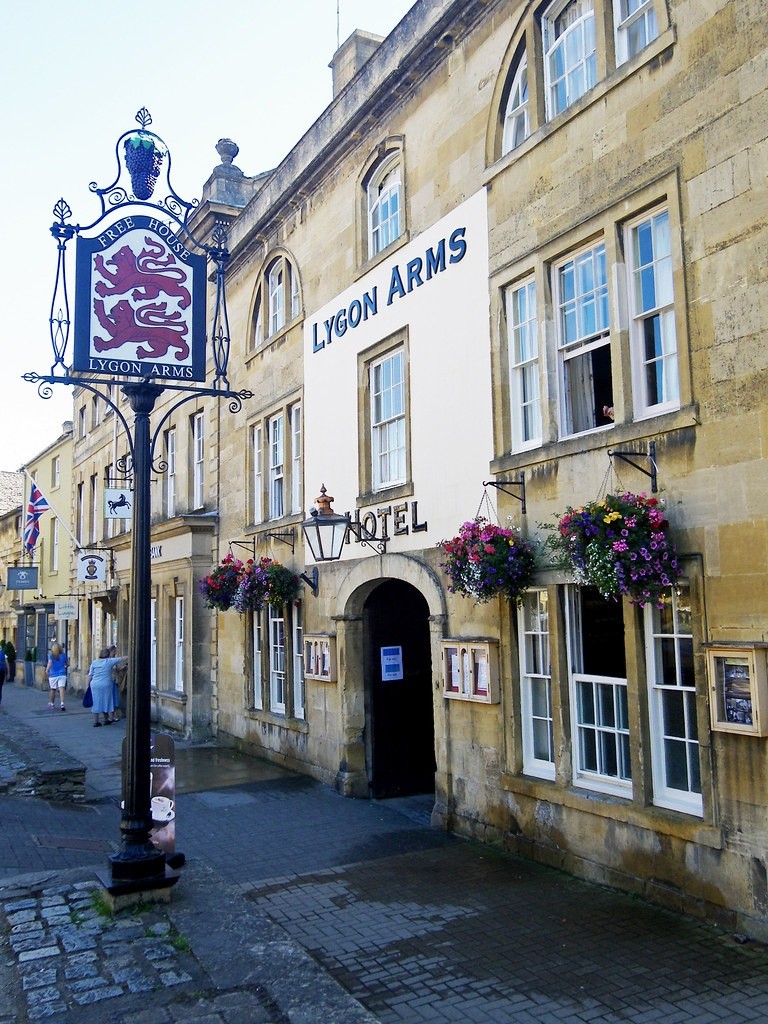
[24,481,50,559]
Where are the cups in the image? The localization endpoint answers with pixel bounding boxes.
[151,796,174,821]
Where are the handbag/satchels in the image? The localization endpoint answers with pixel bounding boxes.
[83,687,93,708]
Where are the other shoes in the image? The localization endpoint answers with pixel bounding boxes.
[114,717,118,721]
[61,705,65,711]
[48,703,54,707]
[108,717,112,721]
[104,721,111,725]
[94,723,101,727]
[6,678,14,682]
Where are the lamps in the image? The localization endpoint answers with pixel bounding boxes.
[300,484,391,563]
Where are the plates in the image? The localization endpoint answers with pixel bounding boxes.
[150,808,175,822]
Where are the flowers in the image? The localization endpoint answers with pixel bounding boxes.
[544,489,688,613]
[427,516,538,611]
[198,552,303,618]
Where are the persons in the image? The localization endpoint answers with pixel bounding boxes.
[46,643,70,711]
[107,645,128,722]
[0,646,7,703]
[0,639,17,682]
[86,649,128,727]
